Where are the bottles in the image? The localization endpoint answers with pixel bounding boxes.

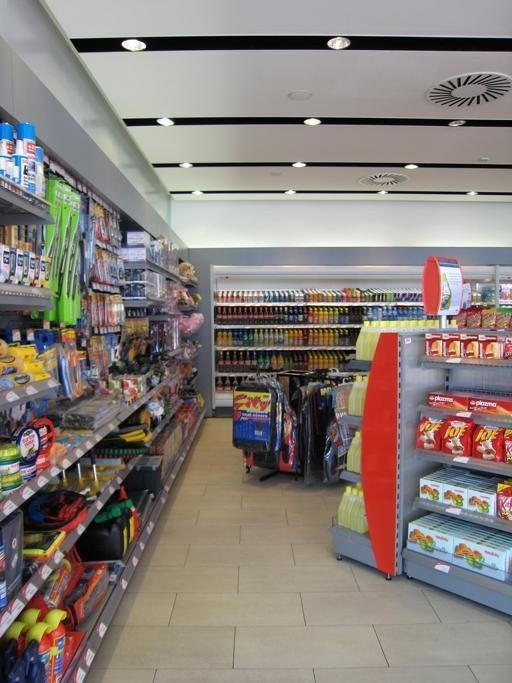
[215,350,353,373]
[216,289,421,302]
[215,376,239,391]
[215,306,425,324]
[216,328,359,348]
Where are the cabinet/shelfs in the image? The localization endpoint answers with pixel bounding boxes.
[330,330,453,581]
[213,291,436,410]
[405,333,512,620]
[1,155,206,681]
